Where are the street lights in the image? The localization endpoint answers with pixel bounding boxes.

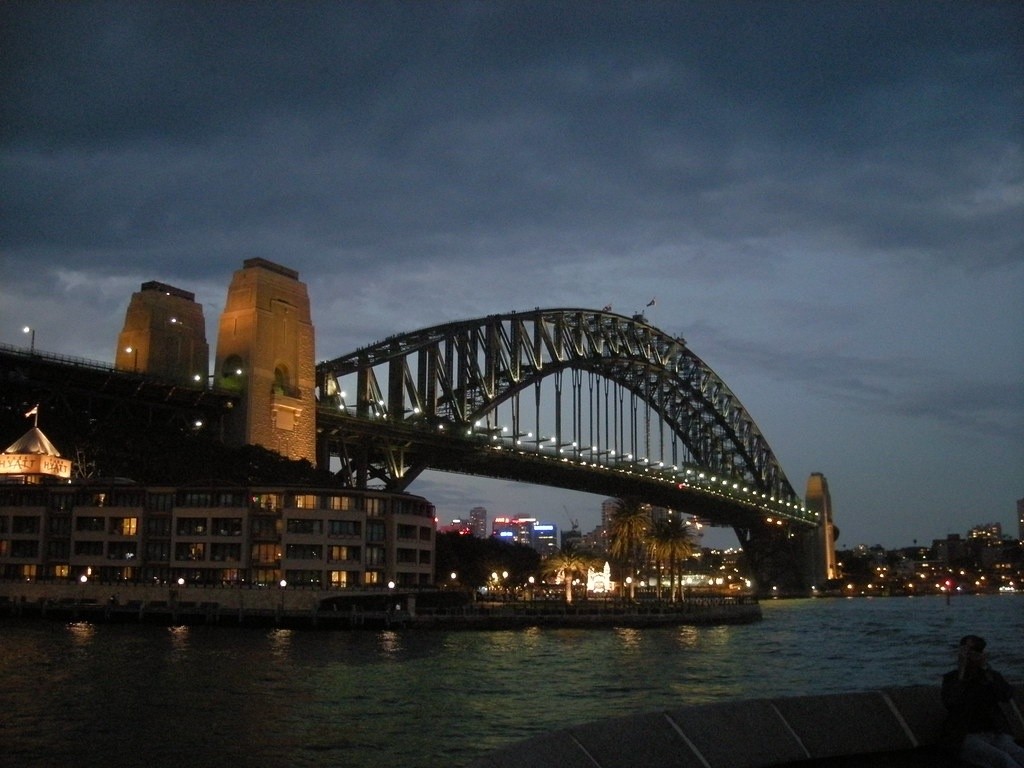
[22,326,36,360]
[126,348,139,374]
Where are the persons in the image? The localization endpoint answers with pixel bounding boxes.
[937,636,1024,768]
